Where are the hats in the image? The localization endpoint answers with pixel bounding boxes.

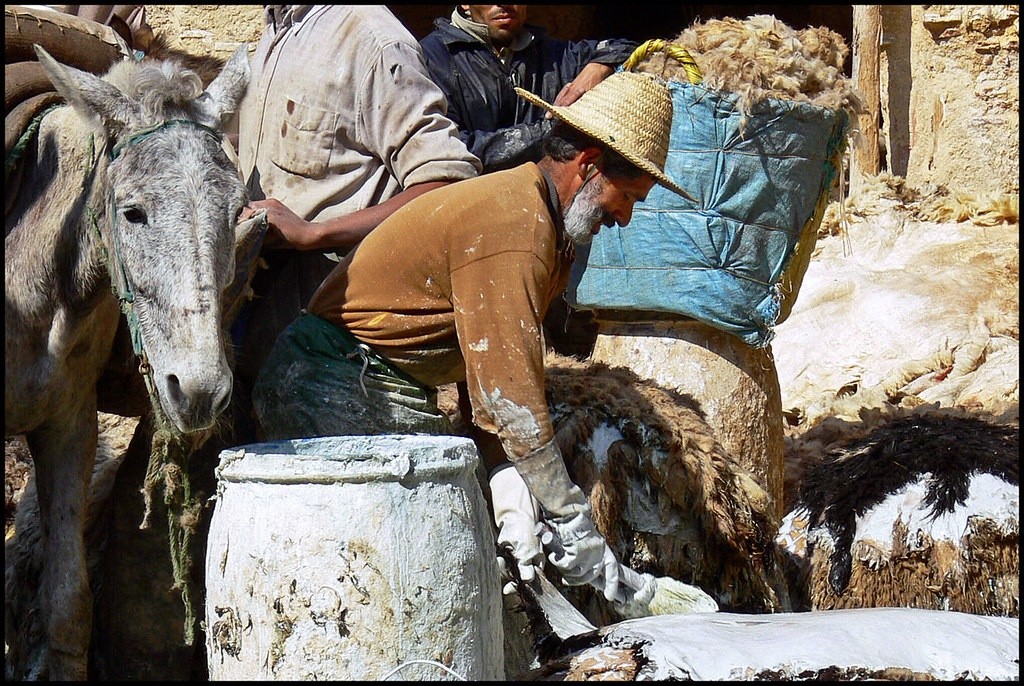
[513,70,700,203]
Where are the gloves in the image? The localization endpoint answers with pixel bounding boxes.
[548,521,620,602]
[484,463,548,595]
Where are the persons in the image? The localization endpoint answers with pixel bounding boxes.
[249,71,703,603]
[416,5,641,174]
[101,6,501,682]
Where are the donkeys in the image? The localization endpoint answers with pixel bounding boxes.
[3,40,251,682]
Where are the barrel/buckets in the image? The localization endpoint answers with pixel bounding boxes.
[577,307,785,530]
[203,433,511,681]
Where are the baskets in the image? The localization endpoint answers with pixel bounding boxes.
[618,37,847,332]
